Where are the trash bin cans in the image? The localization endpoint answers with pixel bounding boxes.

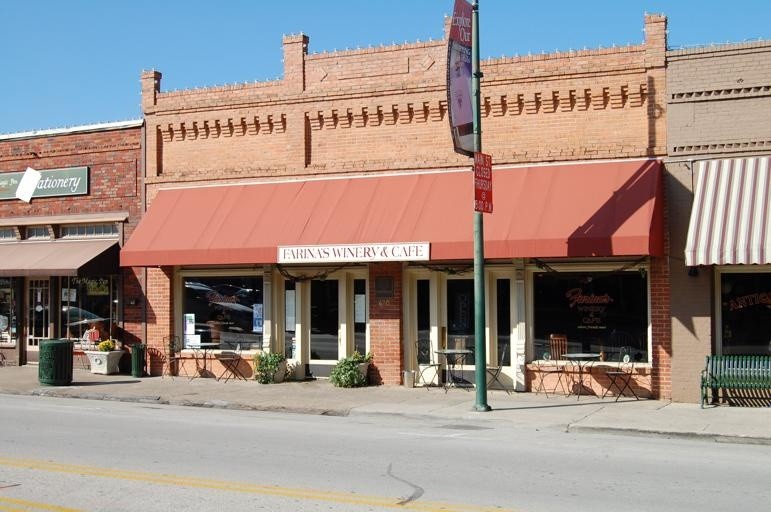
[130,344,148,378]
[38,339,75,386]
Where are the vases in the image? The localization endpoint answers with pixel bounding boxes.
[83,349,125,375]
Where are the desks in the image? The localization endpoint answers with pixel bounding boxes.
[433,348,472,393]
[559,353,600,401]
[185,340,221,382]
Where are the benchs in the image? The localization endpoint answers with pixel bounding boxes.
[698,353,770,409]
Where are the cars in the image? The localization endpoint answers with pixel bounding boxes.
[210,284,244,298]
[28,305,104,339]
[185,281,254,332]
[232,288,263,308]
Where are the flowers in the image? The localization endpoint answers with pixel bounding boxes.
[94,338,114,352]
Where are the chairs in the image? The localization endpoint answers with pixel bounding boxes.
[533,344,566,400]
[486,342,511,396]
[546,333,567,362]
[413,337,445,392]
[604,345,640,403]
[216,342,248,384]
[160,335,189,382]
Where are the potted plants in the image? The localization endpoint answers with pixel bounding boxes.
[330,350,374,389]
[252,351,292,386]
